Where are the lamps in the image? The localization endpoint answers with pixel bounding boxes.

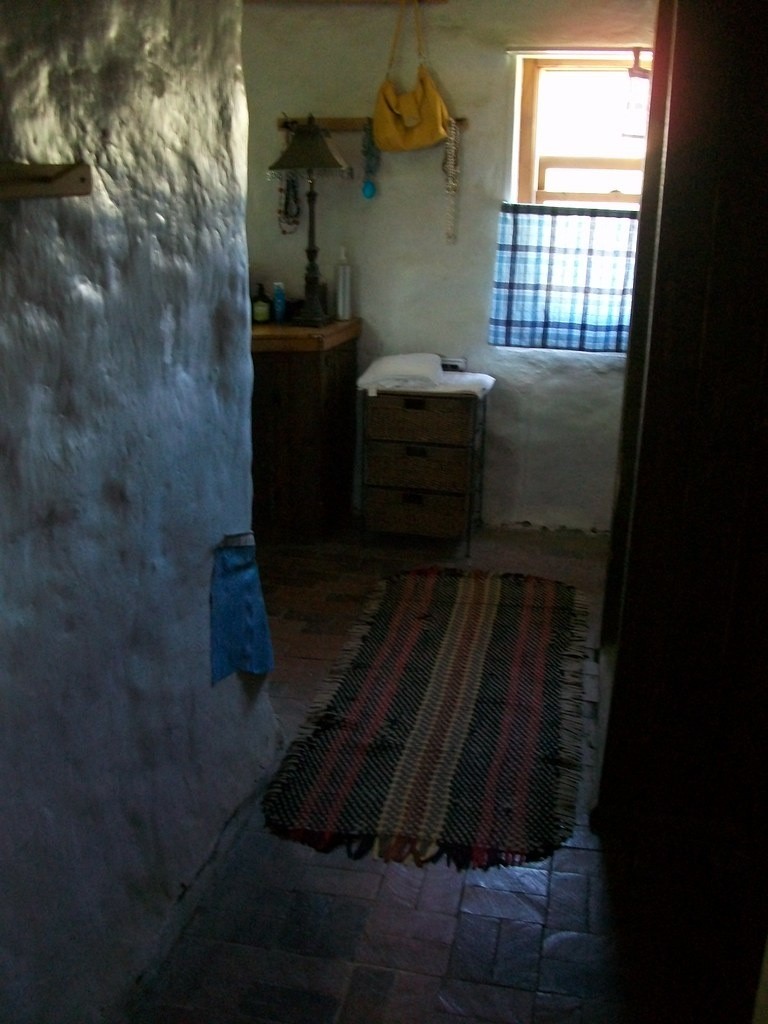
[268,115,343,329]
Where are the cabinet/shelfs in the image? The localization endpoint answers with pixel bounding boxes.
[253,317,362,547]
[352,389,489,559]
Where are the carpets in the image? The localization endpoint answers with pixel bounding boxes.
[261,562,590,871]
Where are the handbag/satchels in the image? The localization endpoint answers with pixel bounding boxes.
[373,65,454,153]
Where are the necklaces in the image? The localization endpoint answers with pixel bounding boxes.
[277,121,301,234]
